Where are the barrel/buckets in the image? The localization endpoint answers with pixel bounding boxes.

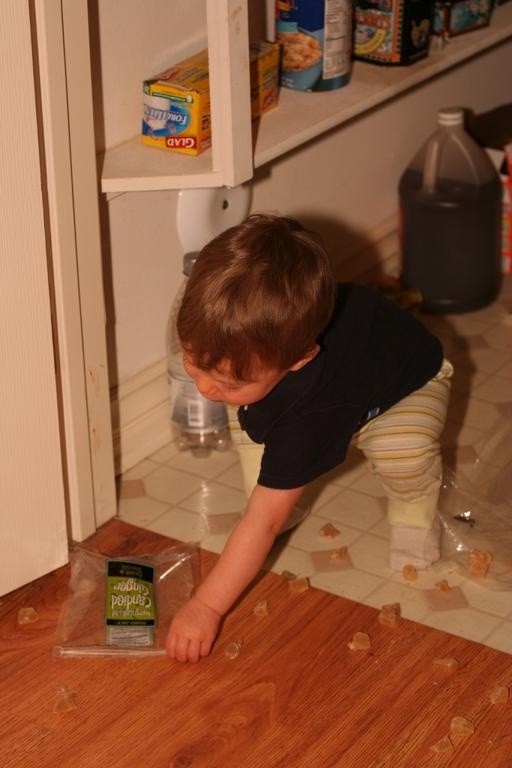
[396,106,499,316]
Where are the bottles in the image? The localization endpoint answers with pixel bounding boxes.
[166,252,233,459]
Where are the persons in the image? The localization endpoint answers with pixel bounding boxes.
[164,213,454,665]
